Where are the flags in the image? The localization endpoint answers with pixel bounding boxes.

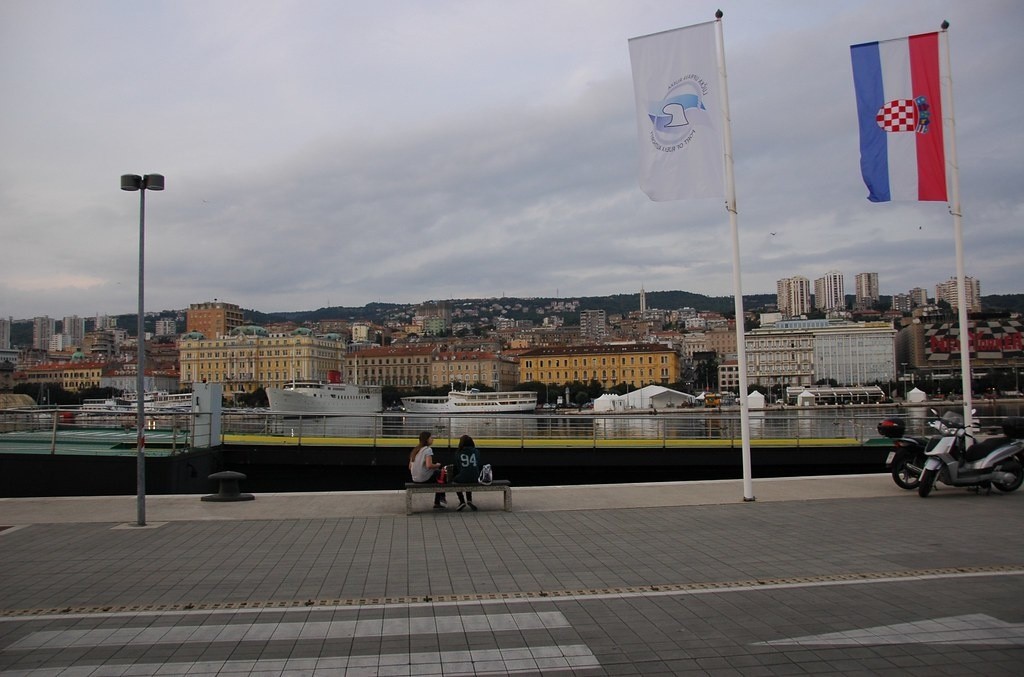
[849,29,949,204]
[628,20,729,202]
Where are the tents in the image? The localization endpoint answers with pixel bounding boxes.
[798,389,815,407]
[747,389,767,409]
[907,387,926,403]
[695,391,707,400]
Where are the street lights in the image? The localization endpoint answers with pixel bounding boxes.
[119,171,166,528]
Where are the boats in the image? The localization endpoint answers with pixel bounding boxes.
[265,354,383,419]
[400,377,539,414]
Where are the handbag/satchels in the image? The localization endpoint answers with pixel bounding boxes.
[478,463,493,485]
[435,466,447,484]
[444,464,454,484]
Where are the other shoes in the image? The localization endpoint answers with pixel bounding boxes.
[432,504,448,510]
[439,501,448,508]
[467,501,478,511]
[455,502,466,511]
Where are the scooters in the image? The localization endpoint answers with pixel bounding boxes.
[875,408,1024,500]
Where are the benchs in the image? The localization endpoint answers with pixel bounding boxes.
[405,480,512,514]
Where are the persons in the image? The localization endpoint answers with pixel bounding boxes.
[408,431,480,511]
